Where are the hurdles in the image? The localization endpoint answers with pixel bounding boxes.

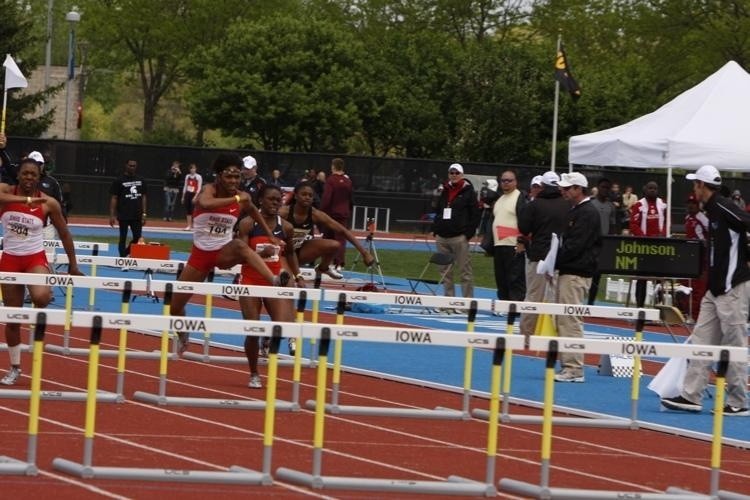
[178,252,333,369]
[0,270,149,405]
[50,309,302,485]
[0,306,67,476]
[274,320,526,500]
[133,278,322,414]
[0,252,55,353]
[496,334,746,500]
[46,253,189,364]
[472,299,661,431]
[41,239,110,311]
[306,288,492,423]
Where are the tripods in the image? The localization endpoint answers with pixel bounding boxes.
[349,234,387,291]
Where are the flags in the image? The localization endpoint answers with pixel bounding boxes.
[3,55,29,93]
[553,38,583,103]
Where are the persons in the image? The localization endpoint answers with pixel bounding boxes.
[431,164,668,383]
[108,158,148,272]
[0,136,84,383]
[661,165,750,418]
[163,155,375,389]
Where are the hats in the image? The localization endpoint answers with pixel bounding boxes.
[28,151,44,163]
[242,155,256,169]
[448,163,464,173]
[685,165,722,185]
[530,171,588,188]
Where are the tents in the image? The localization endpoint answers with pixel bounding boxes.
[569,60,750,238]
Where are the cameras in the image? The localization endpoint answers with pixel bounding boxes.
[365,217,375,224]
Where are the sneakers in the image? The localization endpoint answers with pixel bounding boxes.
[277,270,290,286]
[661,396,703,411]
[710,406,750,416]
[555,366,584,382]
[315,264,343,279]
[0,368,18,385]
[248,337,295,388]
